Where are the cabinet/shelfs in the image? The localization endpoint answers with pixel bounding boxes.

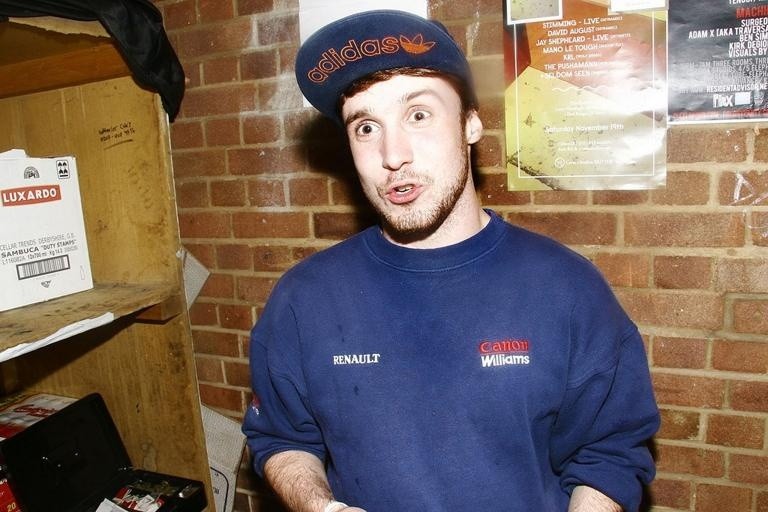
[0,11,219,512]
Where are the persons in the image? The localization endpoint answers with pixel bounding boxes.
[240,9,663,512]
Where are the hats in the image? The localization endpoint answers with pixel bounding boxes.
[295,9,465,121]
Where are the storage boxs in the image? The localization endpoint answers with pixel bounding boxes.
[0,154,95,316]
